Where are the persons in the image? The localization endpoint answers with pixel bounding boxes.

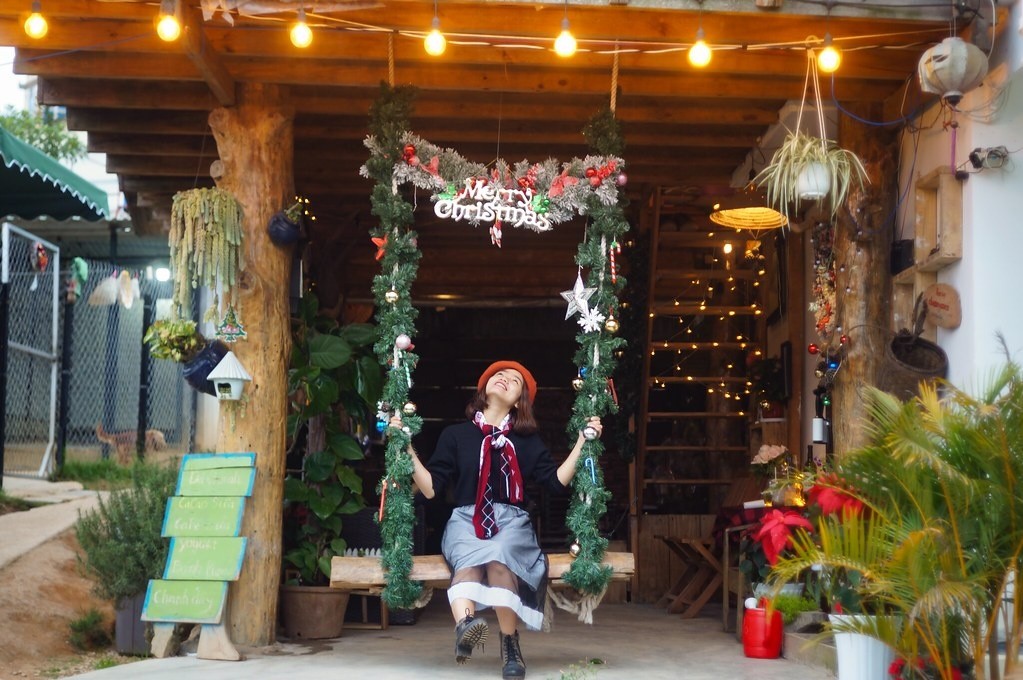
[390,361,603,680]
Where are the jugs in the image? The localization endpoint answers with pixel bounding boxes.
[742,597,783,659]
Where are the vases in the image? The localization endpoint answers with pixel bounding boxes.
[828,614,902,680]
[751,583,804,599]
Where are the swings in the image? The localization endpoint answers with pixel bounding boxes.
[328,36,640,591]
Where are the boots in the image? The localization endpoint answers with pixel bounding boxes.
[454,608,490,665]
[499,629,526,680]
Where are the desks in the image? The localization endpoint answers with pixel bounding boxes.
[712,506,782,543]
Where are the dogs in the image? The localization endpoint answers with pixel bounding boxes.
[97,422,167,467]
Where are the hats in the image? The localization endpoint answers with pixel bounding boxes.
[117,270,134,309]
[478,361,537,407]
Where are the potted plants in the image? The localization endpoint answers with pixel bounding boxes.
[744,121,872,238]
[269,204,303,246]
[143,318,252,396]
[73,453,183,656]
[285,296,381,640]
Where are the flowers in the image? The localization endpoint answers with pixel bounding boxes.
[742,329,1022,680]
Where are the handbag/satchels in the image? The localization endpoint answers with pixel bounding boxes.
[131,272,140,301]
[88,270,117,307]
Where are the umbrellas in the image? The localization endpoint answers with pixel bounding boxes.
[0,126,112,222]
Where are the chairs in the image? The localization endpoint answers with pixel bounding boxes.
[654,463,770,619]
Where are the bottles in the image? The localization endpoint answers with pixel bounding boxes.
[803,445,816,491]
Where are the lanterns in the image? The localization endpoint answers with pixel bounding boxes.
[927,36,989,175]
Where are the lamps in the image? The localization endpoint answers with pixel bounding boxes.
[708,170,788,230]
